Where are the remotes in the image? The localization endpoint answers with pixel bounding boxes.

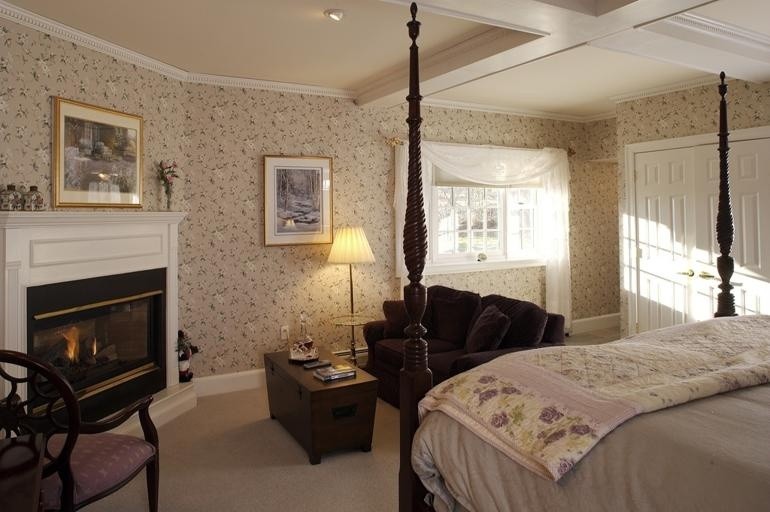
[304,359,332,370]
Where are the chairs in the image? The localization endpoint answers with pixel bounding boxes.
[0,347,160,510]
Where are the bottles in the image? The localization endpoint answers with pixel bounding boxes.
[294,310,314,349]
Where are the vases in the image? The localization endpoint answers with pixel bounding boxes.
[162,183,175,211]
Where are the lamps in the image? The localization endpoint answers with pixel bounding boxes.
[328,227,376,327]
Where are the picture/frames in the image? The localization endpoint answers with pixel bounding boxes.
[49,97,145,209]
[262,155,334,246]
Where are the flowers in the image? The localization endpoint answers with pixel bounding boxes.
[155,158,181,189]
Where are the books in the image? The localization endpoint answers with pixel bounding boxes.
[313,365,356,382]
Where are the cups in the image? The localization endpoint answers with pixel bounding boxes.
[0,183,47,212]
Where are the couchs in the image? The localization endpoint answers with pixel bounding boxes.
[363,283,557,408]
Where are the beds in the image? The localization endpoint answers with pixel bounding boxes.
[410,314,770,508]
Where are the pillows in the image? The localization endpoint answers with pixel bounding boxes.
[465,304,509,355]
[381,298,431,338]
